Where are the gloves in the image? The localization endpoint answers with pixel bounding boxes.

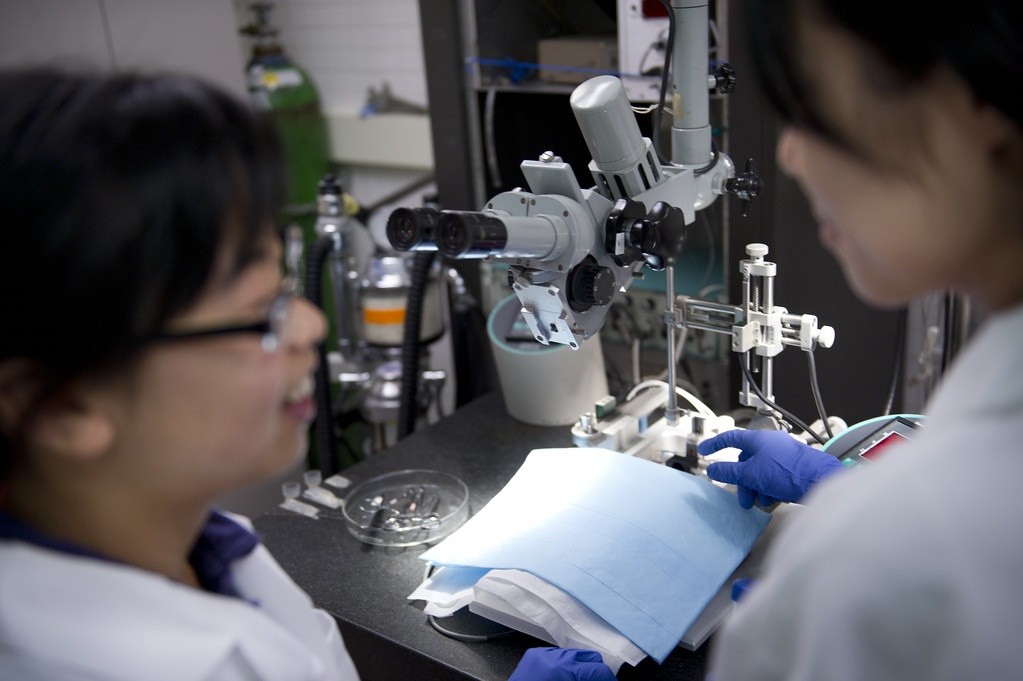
[697,429,847,510]
[508,647,616,681]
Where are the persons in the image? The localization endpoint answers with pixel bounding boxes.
[0,0,1023,681]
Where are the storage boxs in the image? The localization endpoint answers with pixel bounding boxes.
[537,37,618,83]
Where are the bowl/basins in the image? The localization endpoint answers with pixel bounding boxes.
[341,469,469,547]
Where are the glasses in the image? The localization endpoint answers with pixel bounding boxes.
[113,270,300,348]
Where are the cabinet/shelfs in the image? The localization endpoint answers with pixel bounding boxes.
[459,0,733,413]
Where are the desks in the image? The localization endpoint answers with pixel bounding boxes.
[252,387,685,681]
